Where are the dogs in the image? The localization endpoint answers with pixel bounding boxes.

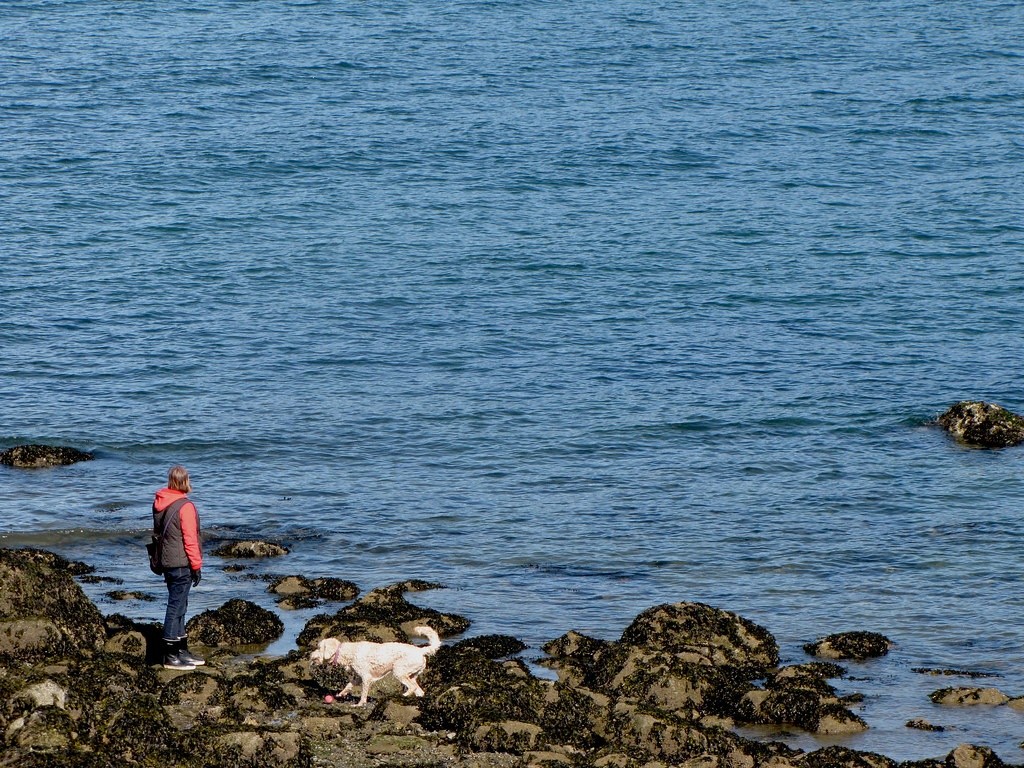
[310,625,441,707]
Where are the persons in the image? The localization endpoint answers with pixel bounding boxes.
[152,466,206,671]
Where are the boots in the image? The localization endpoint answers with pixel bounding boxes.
[162,638,196,670]
[177,634,205,666]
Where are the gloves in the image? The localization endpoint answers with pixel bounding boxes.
[192,569,201,587]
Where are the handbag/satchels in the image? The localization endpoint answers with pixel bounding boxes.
[145,535,163,576]
